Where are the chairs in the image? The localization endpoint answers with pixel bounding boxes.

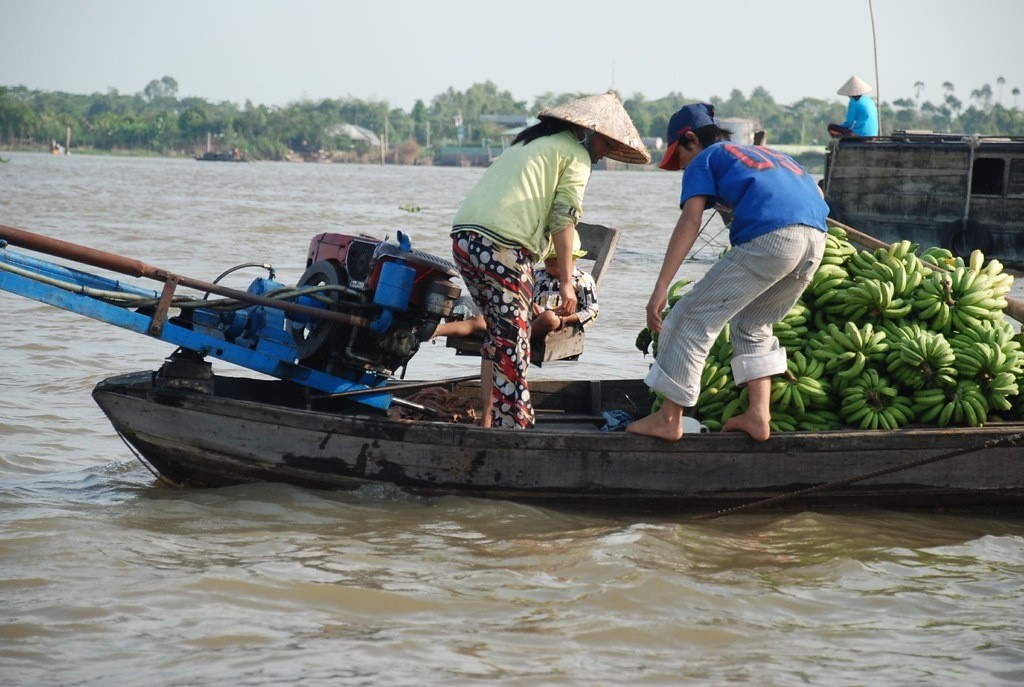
[446,221,623,363]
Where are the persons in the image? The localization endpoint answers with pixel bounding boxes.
[426,228,598,340]
[626,101,830,440]
[451,95,620,429]
[835,75,879,141]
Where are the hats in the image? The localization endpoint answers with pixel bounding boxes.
[537,92,652,165]
[547,229,587,260]
[659,102,716,171]
[837,75,873,96]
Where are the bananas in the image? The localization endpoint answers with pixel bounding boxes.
[636,227,1024,434]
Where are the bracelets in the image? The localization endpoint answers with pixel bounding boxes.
[432,324,439,343]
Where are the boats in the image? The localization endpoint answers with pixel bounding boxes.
[51,140,71,155]
[432,116,539,166]
[1,225,1024,506]
[821,129,1024,269]
[194,151,248,162]
